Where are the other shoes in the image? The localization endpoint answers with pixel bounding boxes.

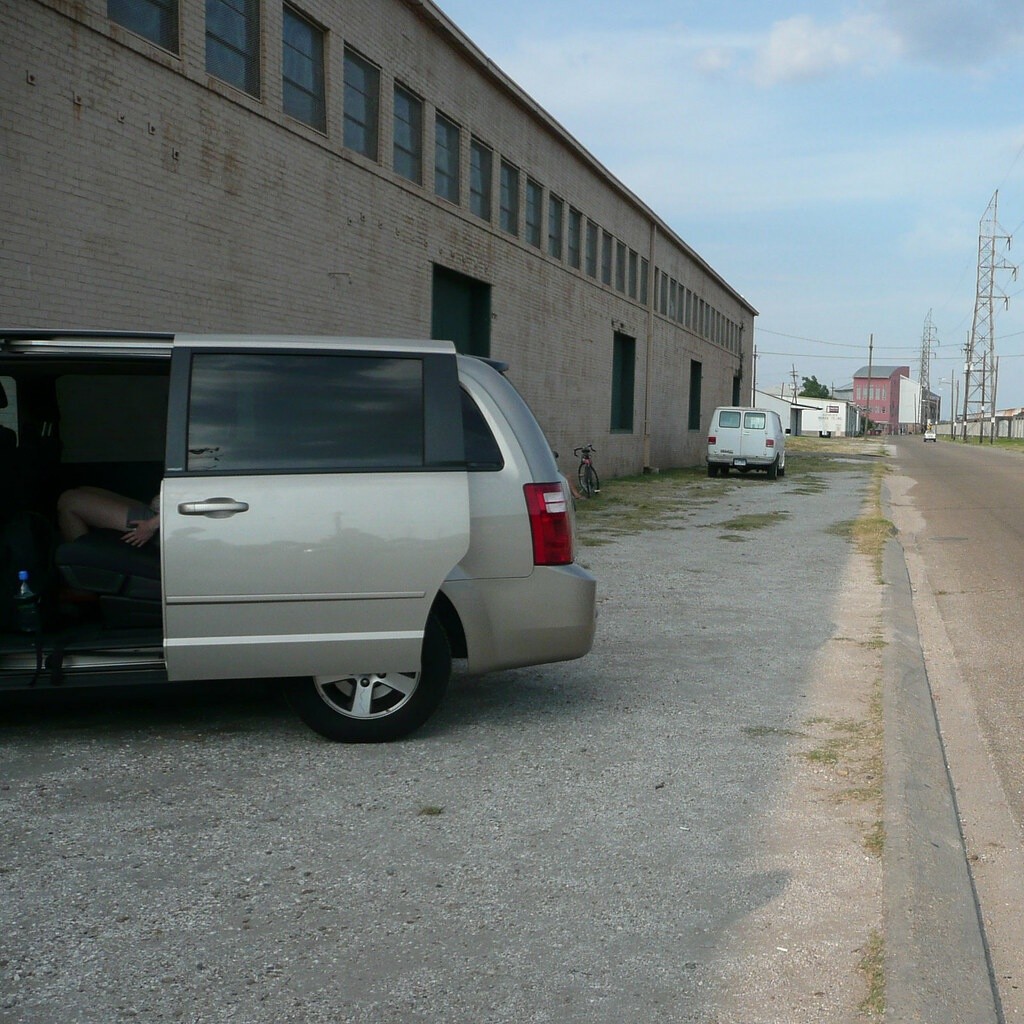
[57,587,97,604]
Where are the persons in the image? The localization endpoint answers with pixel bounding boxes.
[57,485,160,603]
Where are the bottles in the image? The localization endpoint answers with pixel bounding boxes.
[14,570,40,646]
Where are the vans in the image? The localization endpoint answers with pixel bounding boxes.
[924,429,936,442]
[706,406,791,480]
[0,325,601,743]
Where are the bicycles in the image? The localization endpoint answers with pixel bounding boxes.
[574,444,599,498]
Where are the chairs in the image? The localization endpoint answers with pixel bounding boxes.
[57,528,161,600]
